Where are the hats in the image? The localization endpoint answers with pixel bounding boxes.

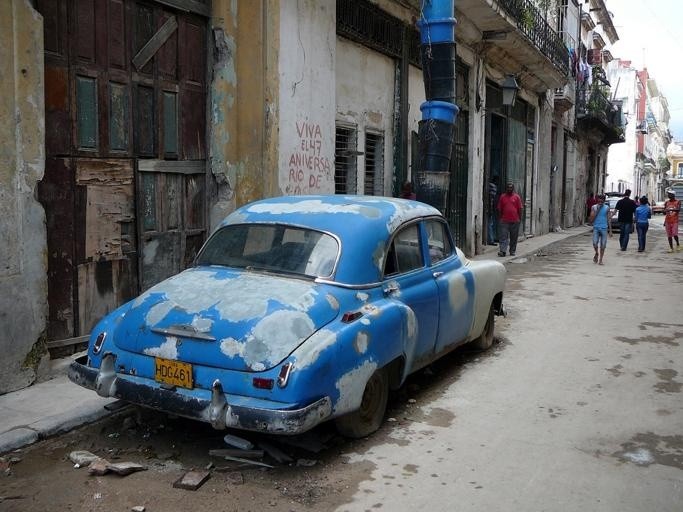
[666,191,676,194]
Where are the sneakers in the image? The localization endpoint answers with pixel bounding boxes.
[498,251,505,257]
[509,249,516,255]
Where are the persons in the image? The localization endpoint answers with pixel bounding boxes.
[590,195,613,265]
[586,192,596,217]
[488,194,498,246]
[611,189,636,251]
[497,183,523,257]
[398,182,415,201]
[634,196,651,252]
[662,190,681,253]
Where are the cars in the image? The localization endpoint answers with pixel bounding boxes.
[68,194,506,438]
[605,198,634,232]
[652,202,666,215]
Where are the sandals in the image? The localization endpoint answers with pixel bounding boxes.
[593,253,598,263]
[676,244,680,251]
[668,248,672,252]
[599,259,604,264]
